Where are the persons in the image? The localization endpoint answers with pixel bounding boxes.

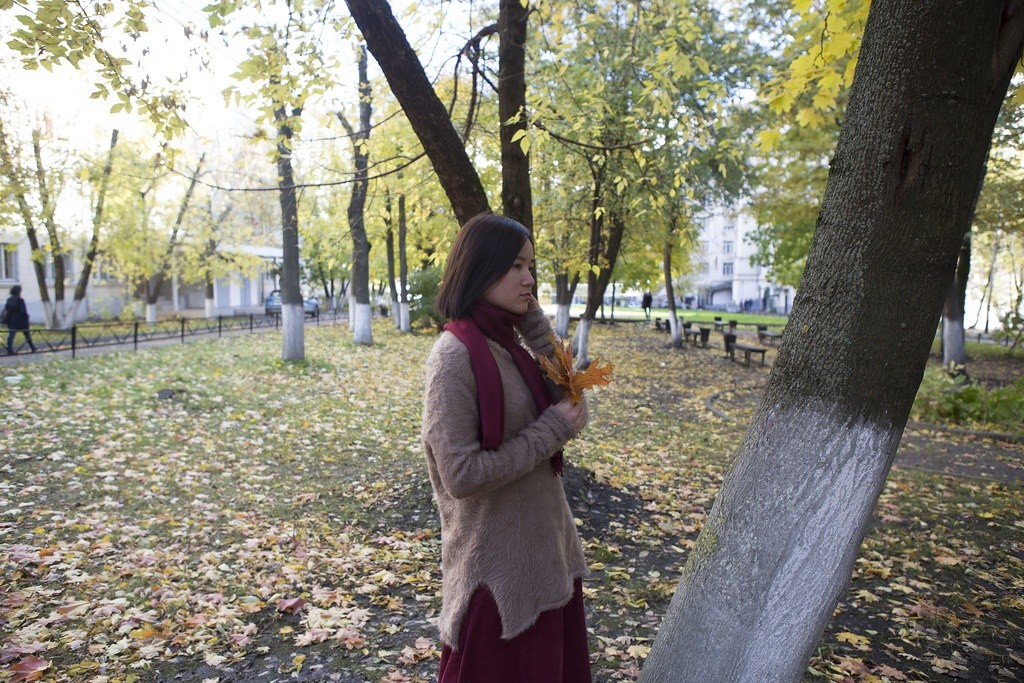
[4,284,38,356]
[416,213,594,683]
[642,289,653,318]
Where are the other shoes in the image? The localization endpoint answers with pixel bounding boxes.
[7,351,16,354]
[33,346,37,353]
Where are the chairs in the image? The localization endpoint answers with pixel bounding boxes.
[655,317,670,333]
[714,316,738,359]
[757,324,767,342]
[682,322,711,348]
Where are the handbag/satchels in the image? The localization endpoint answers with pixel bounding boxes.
[0,309,10,324]
[642,301,646,308]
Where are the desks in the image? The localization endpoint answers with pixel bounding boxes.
[713,321,730,333]
[685,329,701,345]
[658,320,666,331]
[730,343,768,367]
[759,330,783,345]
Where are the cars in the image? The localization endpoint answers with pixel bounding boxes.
[265,289,320,318]
[661,300,690,310]
